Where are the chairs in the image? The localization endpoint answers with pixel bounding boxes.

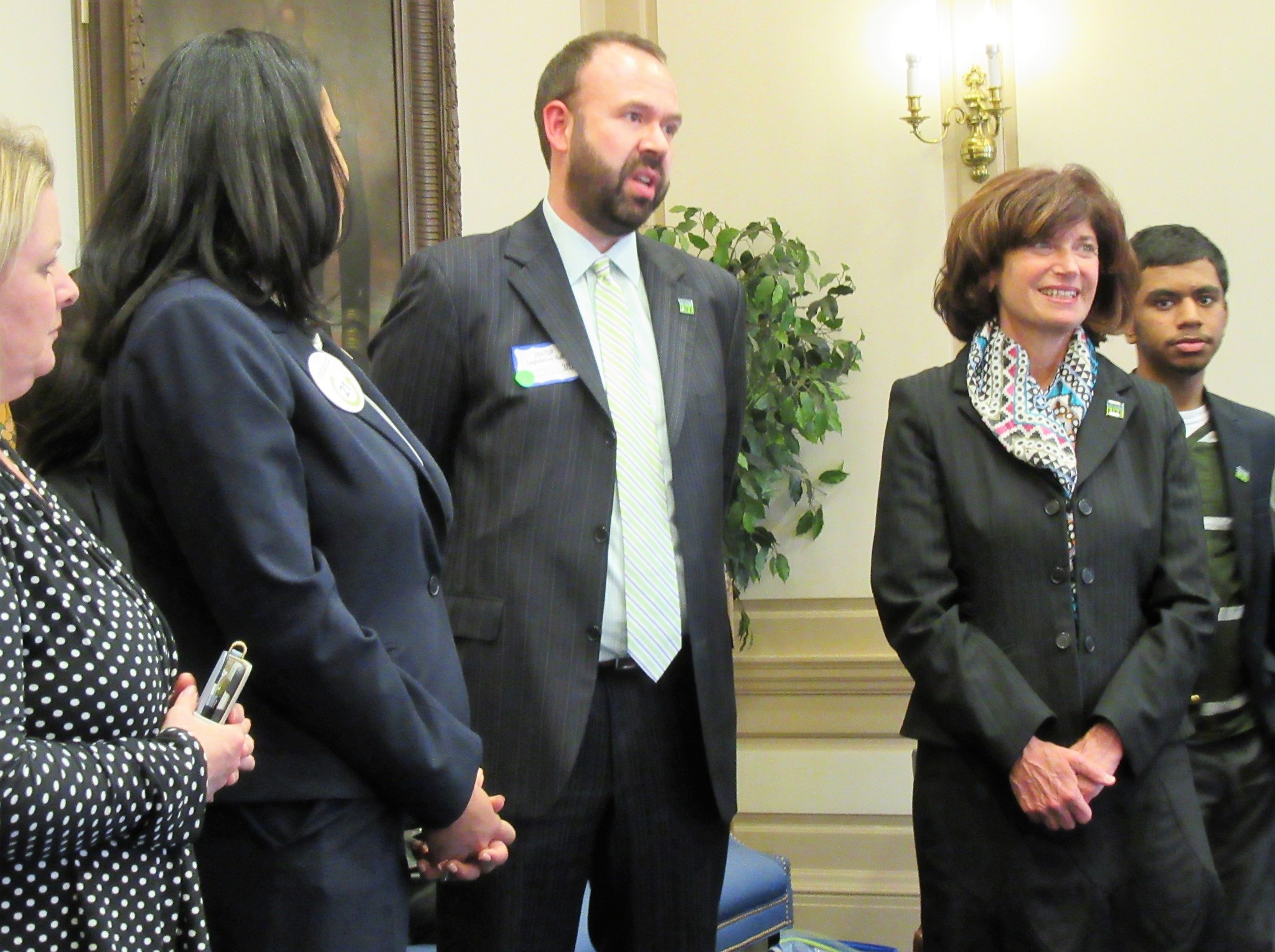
[410,832,793,951]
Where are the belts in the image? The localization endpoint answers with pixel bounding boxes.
[600,656,639,674]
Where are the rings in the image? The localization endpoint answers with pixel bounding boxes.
[436,869,448,886]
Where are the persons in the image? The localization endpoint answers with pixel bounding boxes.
[11,29,515,952]
[871,164,1221,952]
[9,266,133,576]
[1127,224,1275,952]
[366,30,746,952]
[0,117,255,952]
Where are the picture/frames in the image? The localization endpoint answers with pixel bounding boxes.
[73,1,463,377]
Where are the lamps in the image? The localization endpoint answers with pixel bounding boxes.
[905,39,1020,217]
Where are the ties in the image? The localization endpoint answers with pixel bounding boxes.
[589,257,682,685]
